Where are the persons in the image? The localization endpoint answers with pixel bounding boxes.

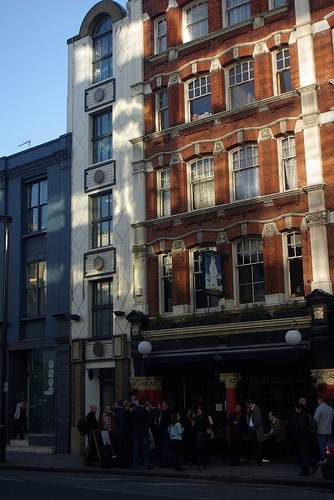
[264,412,287,443]
[181,406,214,470]
[226,404,246,466]
[292,398,313,417]
[294,404,318,476]
[314,396,334,464]
[167,413,185,471]
[87,401,171,469]
[13,400,26,439]
[245,400,264,464]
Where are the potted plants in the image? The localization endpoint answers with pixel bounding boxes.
[146,304,231,331]
[271,301,305,319]
[237,301,271,322]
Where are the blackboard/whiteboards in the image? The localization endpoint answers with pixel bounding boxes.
[87,430,119,460]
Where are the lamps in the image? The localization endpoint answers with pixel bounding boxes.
[285,320,301,345]
[69,314,80,321]
[138,333,154,358]
[113,310,125,316]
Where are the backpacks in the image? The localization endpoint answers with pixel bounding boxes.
[77,417,91,435]
